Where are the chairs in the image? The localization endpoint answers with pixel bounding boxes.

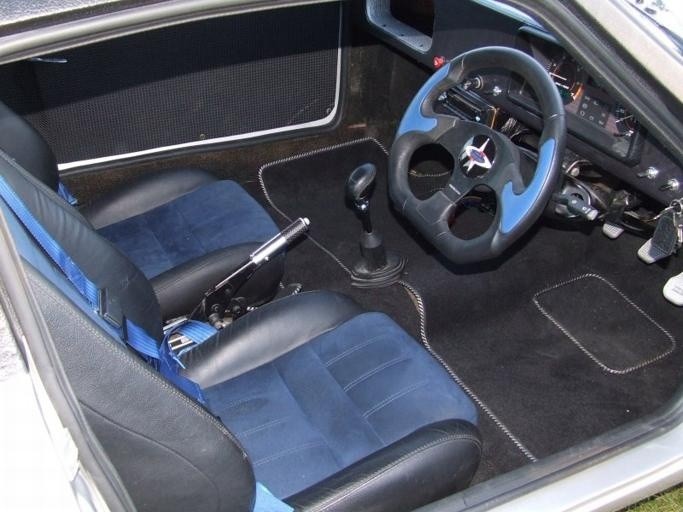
[0,151,481,511]
[0,101,287,314]
